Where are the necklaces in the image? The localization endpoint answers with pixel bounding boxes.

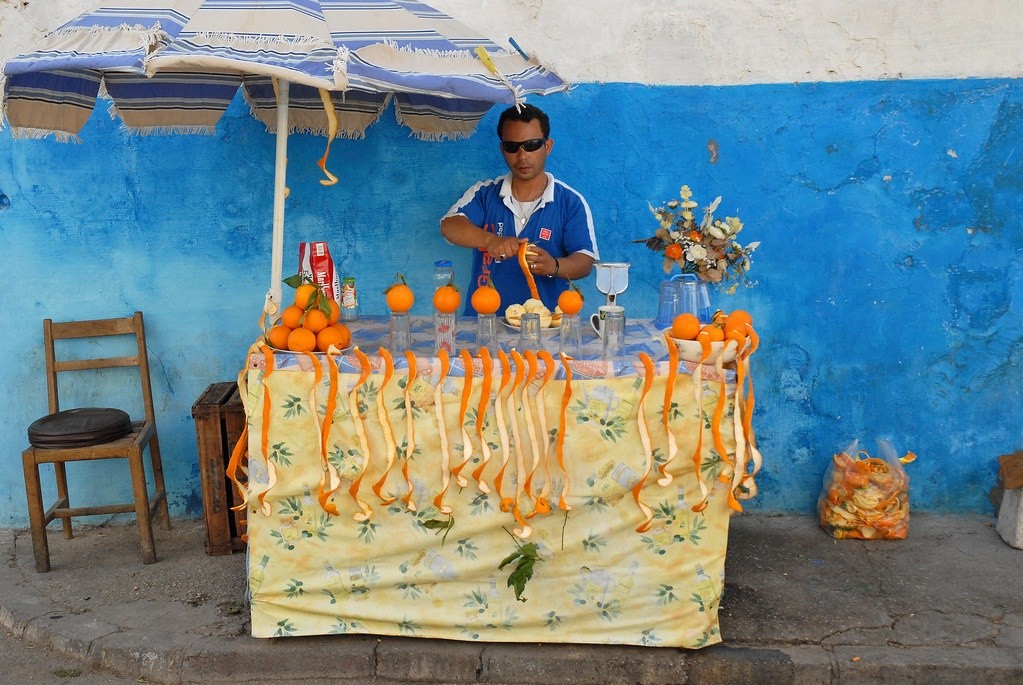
[512,176,547,227]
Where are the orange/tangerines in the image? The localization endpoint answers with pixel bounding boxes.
[266,284,351,353]
[386,283,414,313]
[505,289,583,329]
[433,286,462,313]
[471,285,501,313]
[671,310,752,341]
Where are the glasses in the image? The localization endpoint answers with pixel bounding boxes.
[502,138,546,153]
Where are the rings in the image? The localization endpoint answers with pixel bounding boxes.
[499,254,506,260]
[531,264,537,268]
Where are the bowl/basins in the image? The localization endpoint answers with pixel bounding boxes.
[660,324,752,365]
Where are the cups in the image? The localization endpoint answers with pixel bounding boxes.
[603,313,627,356]
[559,314,582,360]
[432,312,457,357]
[475,313,498,359]
[389,311,412,353]
[519,313,542,355]
[655,278,712,326]
[589,305,625,340]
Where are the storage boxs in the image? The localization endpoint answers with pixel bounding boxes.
[190,378,246,556]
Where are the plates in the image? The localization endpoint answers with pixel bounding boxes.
[263,337,356,355]
[499,317,562,333]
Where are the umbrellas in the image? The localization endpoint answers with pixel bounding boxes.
[3,0,572,327]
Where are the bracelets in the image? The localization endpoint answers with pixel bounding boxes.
[547,256,559,279]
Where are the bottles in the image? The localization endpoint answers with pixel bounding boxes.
[340,278,361,322]
[432,260,456,291]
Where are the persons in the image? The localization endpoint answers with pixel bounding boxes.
[439,103,600,318]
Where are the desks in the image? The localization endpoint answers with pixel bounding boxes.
[247,316,751,651]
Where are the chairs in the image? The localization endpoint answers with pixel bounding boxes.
[21,311,172,573]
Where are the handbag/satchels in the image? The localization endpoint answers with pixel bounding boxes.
[817,438,911,539]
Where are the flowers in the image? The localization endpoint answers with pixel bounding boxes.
[630,184,762,296]
